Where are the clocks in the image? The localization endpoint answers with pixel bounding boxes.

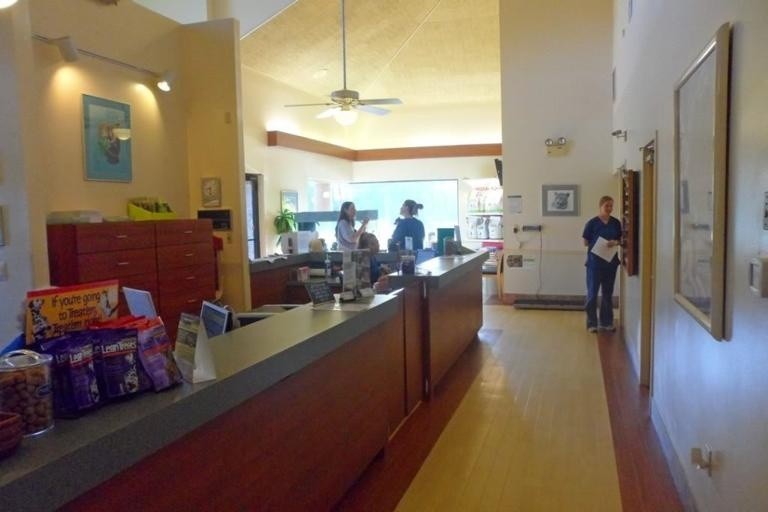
[200,177,222,208]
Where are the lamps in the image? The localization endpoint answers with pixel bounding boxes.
[545,137,568,156]
[335,106,358,124]
[32,35,181,91]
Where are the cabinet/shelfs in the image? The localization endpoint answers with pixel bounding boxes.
[45,217,217,350]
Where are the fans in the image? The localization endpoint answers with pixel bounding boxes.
[284,0,404,119]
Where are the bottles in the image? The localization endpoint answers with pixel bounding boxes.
[324,254,332,278]
[0,352,54,440]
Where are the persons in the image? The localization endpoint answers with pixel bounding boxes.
[358,231,391,285]
[334,200,369,252]
[387,199,425,252]
[580,195,623,334]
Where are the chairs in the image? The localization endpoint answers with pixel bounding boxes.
[482,249,504,300]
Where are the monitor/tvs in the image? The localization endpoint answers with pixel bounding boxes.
[415,248,436,265]
[200,300,232,338]
[494,159,503,186]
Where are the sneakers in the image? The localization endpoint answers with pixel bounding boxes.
[587,326,616,333]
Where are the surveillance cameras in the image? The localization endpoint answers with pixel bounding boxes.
[611,130,622,137]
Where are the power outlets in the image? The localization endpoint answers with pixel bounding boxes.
[703,442,712,479]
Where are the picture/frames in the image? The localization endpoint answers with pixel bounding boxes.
[671,20,732,342]
[542,185,578,217]
[82,94,133,182]
[281,190,299,213]
